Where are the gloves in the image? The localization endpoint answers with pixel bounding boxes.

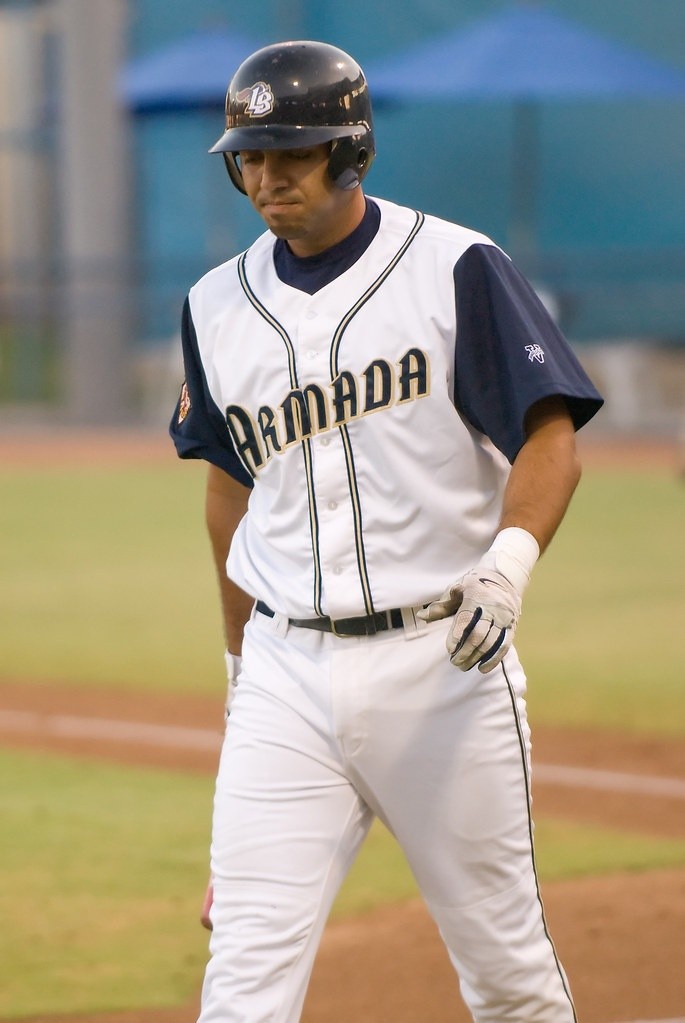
[417,551,530,674]
[224,648,242,726]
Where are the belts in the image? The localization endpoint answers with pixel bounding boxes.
[255,600,441,637]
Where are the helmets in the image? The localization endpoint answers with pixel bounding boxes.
[208,40,377,197]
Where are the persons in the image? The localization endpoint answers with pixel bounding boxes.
[166,41,604,1023]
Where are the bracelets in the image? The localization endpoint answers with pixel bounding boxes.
[489,527,540,571]
